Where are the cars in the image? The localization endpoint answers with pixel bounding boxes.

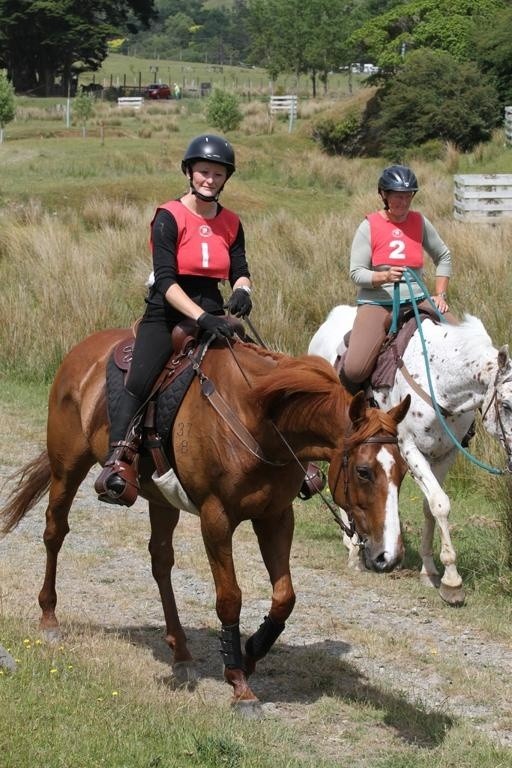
[141,82,170,99]
[78,81,104,93]
[339,62,382,75]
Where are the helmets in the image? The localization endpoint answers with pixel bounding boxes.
[182,135,235,174]
[379,165,419,192]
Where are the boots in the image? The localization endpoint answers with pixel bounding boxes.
[106,388,142,488]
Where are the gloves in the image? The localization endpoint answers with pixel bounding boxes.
[224,288,252,318]
[197,312,234,340]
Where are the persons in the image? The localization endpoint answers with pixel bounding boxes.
[108,137,321,492]
[174,84,180,101]
[335,164,450,391]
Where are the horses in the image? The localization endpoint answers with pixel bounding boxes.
[0,327,412,720]
[306,303,512,606]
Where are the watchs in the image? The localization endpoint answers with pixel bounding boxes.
[437,292,448,301]
[236,285,252,294]
